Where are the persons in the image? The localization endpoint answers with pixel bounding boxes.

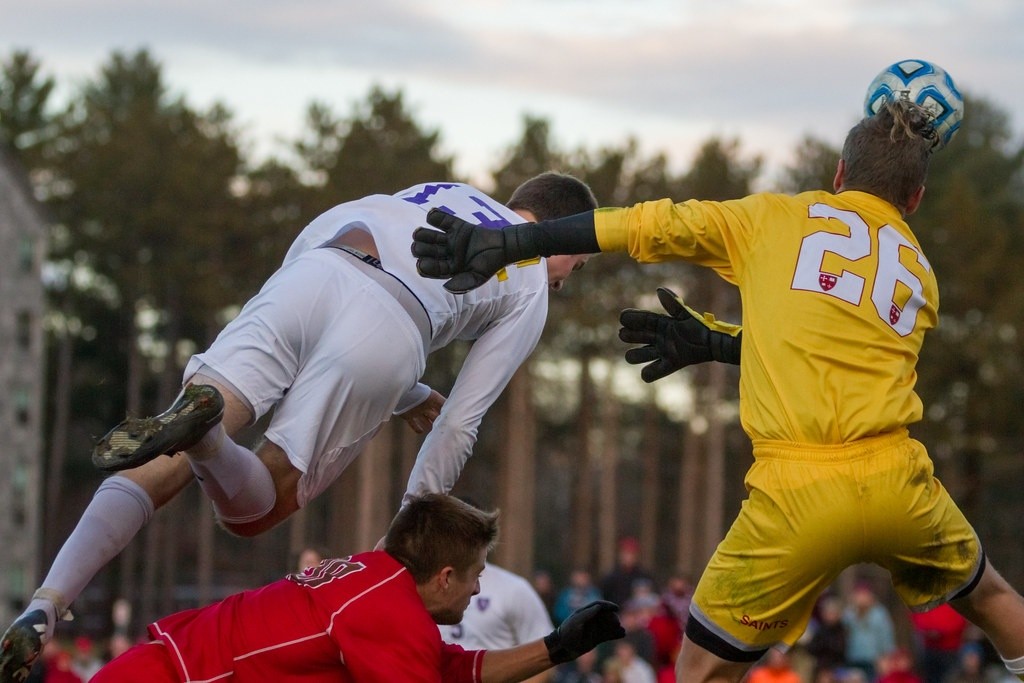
[410,97,1024,683]
[0,535,1024,683]
[0,171,600,683]
[89,492,625,683]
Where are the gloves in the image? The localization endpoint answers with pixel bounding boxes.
[618,288,743,383]
[544,601,627,665]
[411,208,537,294]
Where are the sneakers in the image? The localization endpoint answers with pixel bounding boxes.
[0,608,48,683]
[91,382,226,472]
